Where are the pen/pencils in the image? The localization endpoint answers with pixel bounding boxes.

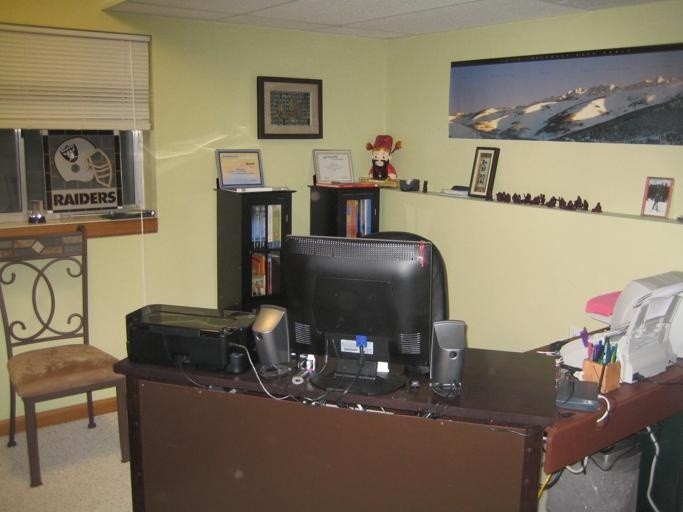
[588,335,618,366]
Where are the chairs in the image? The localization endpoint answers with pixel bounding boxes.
[360,231,449,323]
[0,224,131,488]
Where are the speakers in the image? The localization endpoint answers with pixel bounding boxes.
[431,321,466,398]
[252,305,292,380]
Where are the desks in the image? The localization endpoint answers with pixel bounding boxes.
[113,327,556,512]
[523,326,683,512]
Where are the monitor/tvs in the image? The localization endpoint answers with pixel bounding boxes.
[279,233,433,396]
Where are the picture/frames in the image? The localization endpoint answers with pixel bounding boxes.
[257,76,322,139]
[468,147,500,198]
[312,149,353,184]
[640,176,675,219]
[215,149,265,190]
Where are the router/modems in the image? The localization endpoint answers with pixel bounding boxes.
[554,379,599,413]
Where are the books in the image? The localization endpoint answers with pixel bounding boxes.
[250,204,283,298]
[346,199,372,238]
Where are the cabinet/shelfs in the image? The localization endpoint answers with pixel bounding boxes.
[307,185,381,239]
[215,189,297,312]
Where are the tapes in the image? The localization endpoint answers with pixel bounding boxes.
[406,179,414,185]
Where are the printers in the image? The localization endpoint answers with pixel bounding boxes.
[125,303,259,373]
